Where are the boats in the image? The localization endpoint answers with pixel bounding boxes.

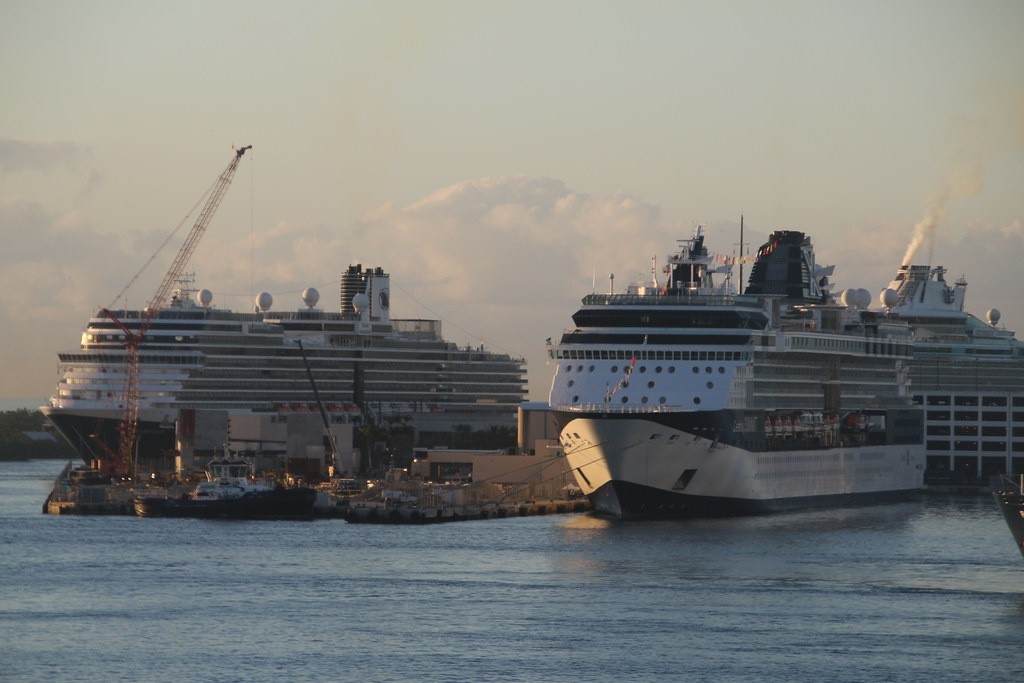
[37,399,590,526]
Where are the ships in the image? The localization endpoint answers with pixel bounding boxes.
[547,224,1024,527]
[38,260,532,487]
